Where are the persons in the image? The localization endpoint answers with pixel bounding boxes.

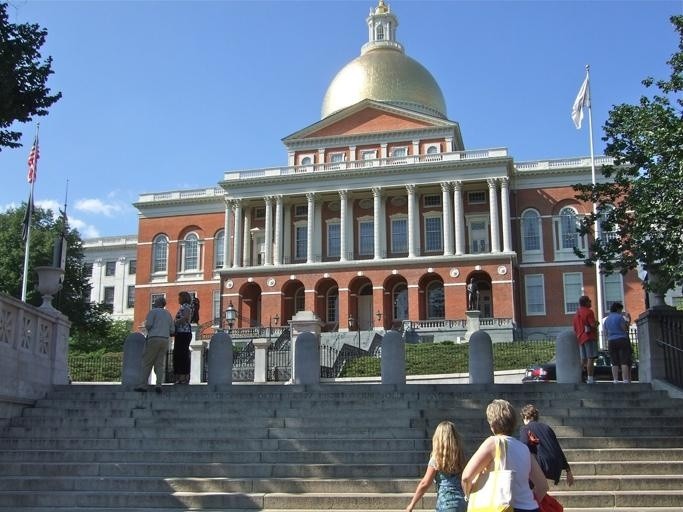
[573,296,600,384]
[603,303,632,383]
[191,293,200,324]
[406,421,467,512]
[134,296,175,392]
[272,363,282,382]
[519,404,575,488]
[174,292,192,384]
[460,398,549,512]
[467,278,479,310]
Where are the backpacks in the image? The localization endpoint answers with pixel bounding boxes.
[520,422,560,485]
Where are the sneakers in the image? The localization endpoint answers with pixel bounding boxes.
[584,380,598,385]
[133,384,163,392]
[612,380,631,384]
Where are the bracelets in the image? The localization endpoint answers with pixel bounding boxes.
[566,470,571,472]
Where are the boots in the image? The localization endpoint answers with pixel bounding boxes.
[174,374,190,385]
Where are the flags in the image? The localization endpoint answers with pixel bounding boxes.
[27,139,40,183]
[571,78,591,130]
[21,193,31,241]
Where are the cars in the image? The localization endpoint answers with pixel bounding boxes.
[522,347,639,385]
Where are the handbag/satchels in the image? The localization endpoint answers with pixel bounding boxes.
[585,325,592,333]
[466,470,517,512]
[527,431,539,448]
